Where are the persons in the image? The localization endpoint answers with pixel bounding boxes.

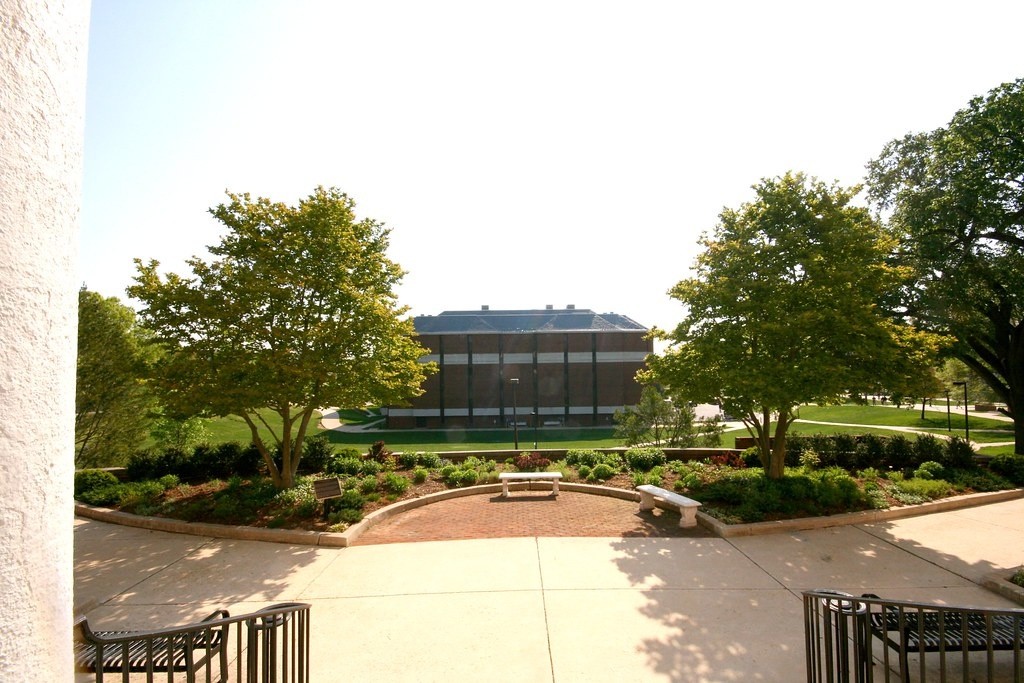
[878,393,887,405]
[956,400,961,409]
[929,397,933,406]
[872,397,876,406]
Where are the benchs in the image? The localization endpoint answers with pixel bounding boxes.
[636,485,701,528]
[862,593,1024,683]
[74,605,230,683]
[499,472,563,496]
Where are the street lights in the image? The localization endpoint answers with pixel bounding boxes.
[953,382,970,445]
